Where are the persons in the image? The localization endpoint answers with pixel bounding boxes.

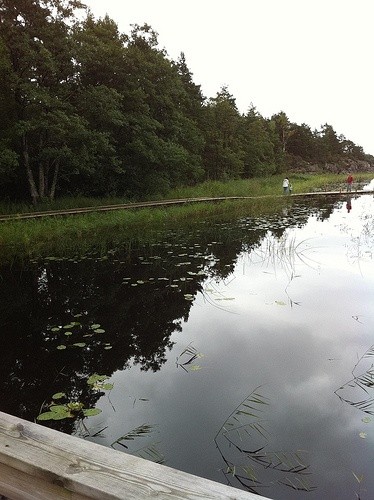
[345,174,353,193]
[289,184,292,195]
[346,197,351,213]
[282,177,289,195]
